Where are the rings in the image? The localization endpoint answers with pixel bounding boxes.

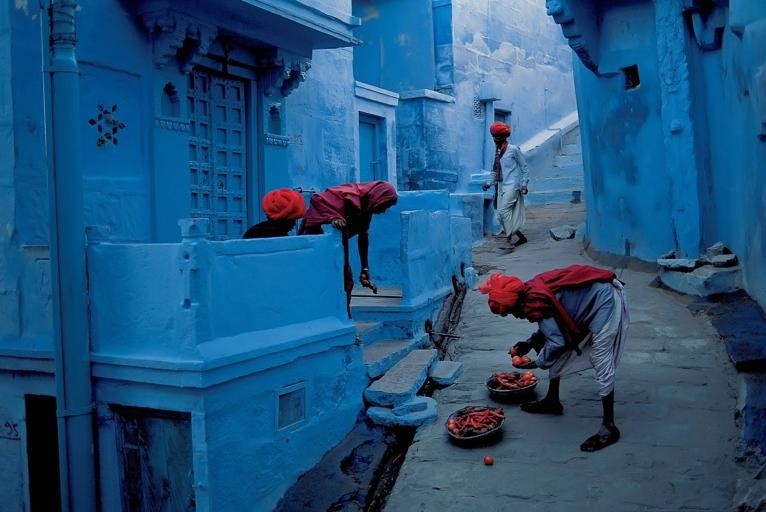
[334,220,339,224]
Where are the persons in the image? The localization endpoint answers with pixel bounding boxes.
[297,180,397,348]
[478,264,631,452]
[242,188,308,240]
[482,121,530,250]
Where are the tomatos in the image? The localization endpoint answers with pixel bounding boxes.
[484,455,494,466]
[509,346,530,364]
[524,372,537,386]
[447,418,456,431]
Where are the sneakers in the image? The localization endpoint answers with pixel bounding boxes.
[521,402,563,414]
[581,427,620,451]
[499,237,527,249]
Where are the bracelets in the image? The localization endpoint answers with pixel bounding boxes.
[361,268,369,271]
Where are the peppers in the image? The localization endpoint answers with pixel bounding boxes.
[458,406,505,432]
[494,371,524,390]
